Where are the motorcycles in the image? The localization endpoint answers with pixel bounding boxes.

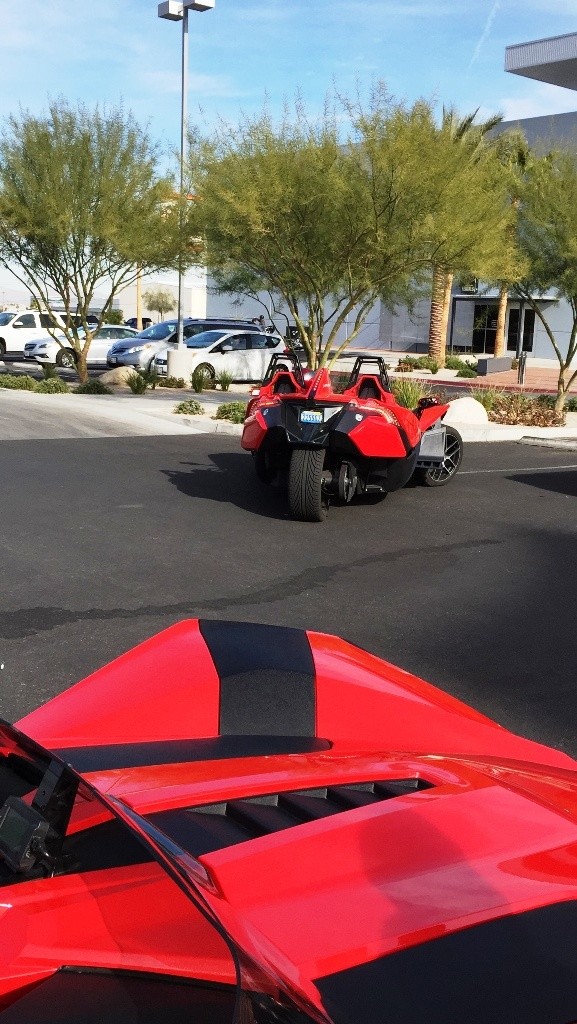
[241,349,465,522]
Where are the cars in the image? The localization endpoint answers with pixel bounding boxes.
[146,328,297,385]
[25,323,143,370]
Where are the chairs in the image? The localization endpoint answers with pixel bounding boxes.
[265,336,275,348]
[262,353,306,394]
[239,337,245,348]
[342,356,392,401]
[100,329,110,339]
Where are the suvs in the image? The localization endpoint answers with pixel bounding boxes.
[0,309,90,362]
[106,316,265,375]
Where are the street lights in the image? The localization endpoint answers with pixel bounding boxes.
[157,0,216,387]
